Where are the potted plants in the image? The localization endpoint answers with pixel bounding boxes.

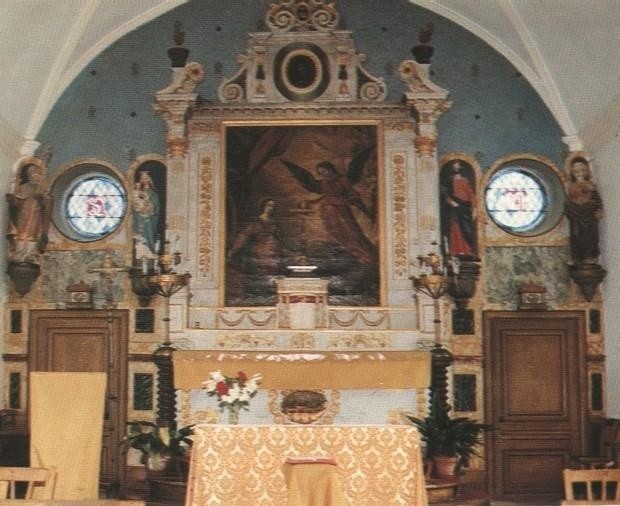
[406,393,497,477]
[116,420,195,506]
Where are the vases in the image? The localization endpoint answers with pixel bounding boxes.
[230,409,239,424]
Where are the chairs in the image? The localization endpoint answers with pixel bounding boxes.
[562,465,619,506]
[1,468,56,506]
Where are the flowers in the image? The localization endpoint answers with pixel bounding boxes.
[202,369,264,414]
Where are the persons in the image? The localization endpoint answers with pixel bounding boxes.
[9,165,53,266]
[437,159,479,260]
[86,194,106,231]
[131,166,161,276]
[563,158,604,265]
[298,163,378,264]
[133,182,148,212]
[225,198,296,304]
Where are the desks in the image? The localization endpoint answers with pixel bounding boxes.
[190,424,425,506]
